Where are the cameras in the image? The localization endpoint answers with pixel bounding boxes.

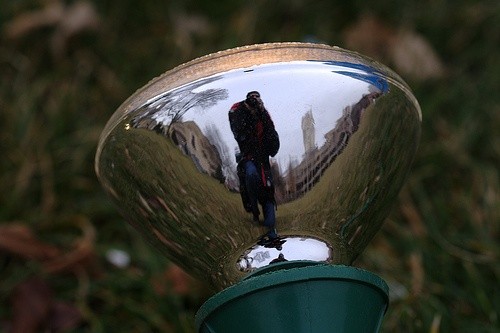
[243,94,259,111]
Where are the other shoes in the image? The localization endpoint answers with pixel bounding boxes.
[253,214,259,225]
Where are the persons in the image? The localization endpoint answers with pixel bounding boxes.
[227,90,283,250]
